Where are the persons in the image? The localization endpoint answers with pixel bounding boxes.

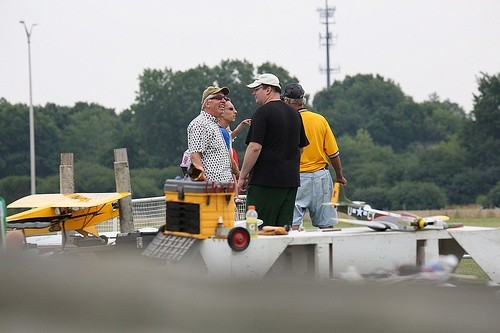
[237,74,310,230]
[187,86,235,184]
[280,83,347,230]
[179,98,252,195]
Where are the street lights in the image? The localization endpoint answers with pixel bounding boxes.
[18,20,39,196]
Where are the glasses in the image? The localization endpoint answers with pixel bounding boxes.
[209,95,228,101]
[224,107,236,111]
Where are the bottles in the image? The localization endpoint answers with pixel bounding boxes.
[215,216,223,236]
[246,206,258,238]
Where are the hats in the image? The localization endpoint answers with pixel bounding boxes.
[201,86,229,103]
[281,84,305,98]
[247,73,281,88]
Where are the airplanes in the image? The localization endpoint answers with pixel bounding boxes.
[328,182,463,233]
[2,188,135,243]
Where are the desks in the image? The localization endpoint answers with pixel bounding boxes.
[142,226,500,281]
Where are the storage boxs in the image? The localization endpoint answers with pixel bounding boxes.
[164,191,236,241]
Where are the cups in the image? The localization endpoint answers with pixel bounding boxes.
[136,236,143,248]
